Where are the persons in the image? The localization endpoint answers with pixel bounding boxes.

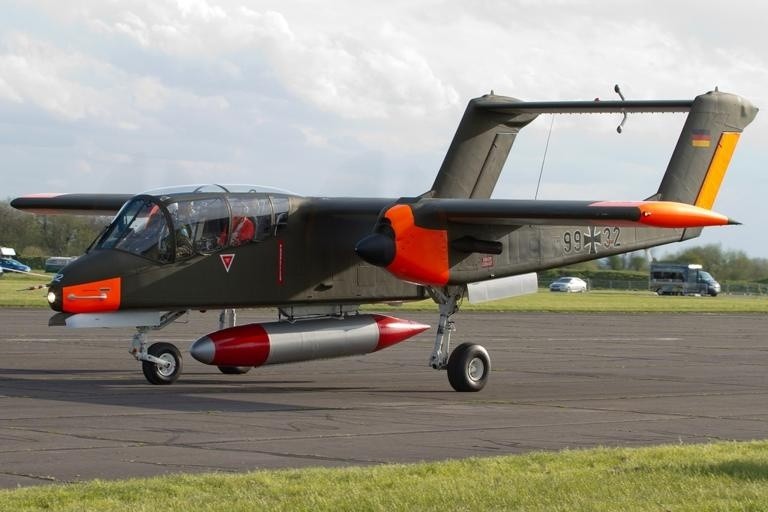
[219,201,254,247]
[158,196,189,249]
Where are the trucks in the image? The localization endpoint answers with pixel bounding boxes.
[648,262,721,297]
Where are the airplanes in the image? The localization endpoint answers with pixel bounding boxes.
[10,86,761,393]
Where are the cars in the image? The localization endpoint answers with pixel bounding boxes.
[0,257,31,274]
[549,276,588,293]
